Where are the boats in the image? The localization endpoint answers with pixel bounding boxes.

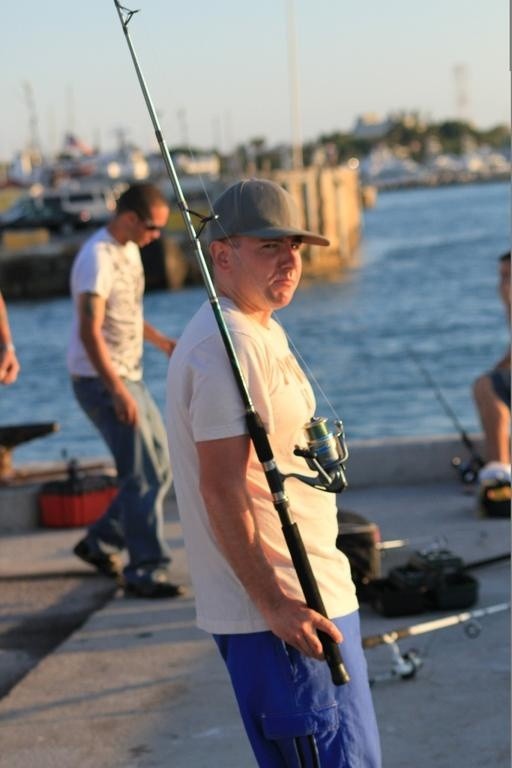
[0,144,378,300]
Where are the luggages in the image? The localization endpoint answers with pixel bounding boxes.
[336,509,446,602]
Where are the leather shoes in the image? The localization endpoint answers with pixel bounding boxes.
[73,537,184,599]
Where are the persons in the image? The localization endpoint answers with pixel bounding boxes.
[0,292,20,385]
[69,177,184,598]
[168,178,380,768]
[474,253,512,480]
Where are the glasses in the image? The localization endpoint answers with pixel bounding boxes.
[144,219,160,231]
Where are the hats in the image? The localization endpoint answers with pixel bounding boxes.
[208,178,330,246]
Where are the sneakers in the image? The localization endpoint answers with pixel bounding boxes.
[478,461,511,483]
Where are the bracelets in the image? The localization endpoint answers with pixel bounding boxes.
[0,344,16,353]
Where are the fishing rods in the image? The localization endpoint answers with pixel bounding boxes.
[113,1,351,686]
[362,603,511,677]
[402,342,486,484]
